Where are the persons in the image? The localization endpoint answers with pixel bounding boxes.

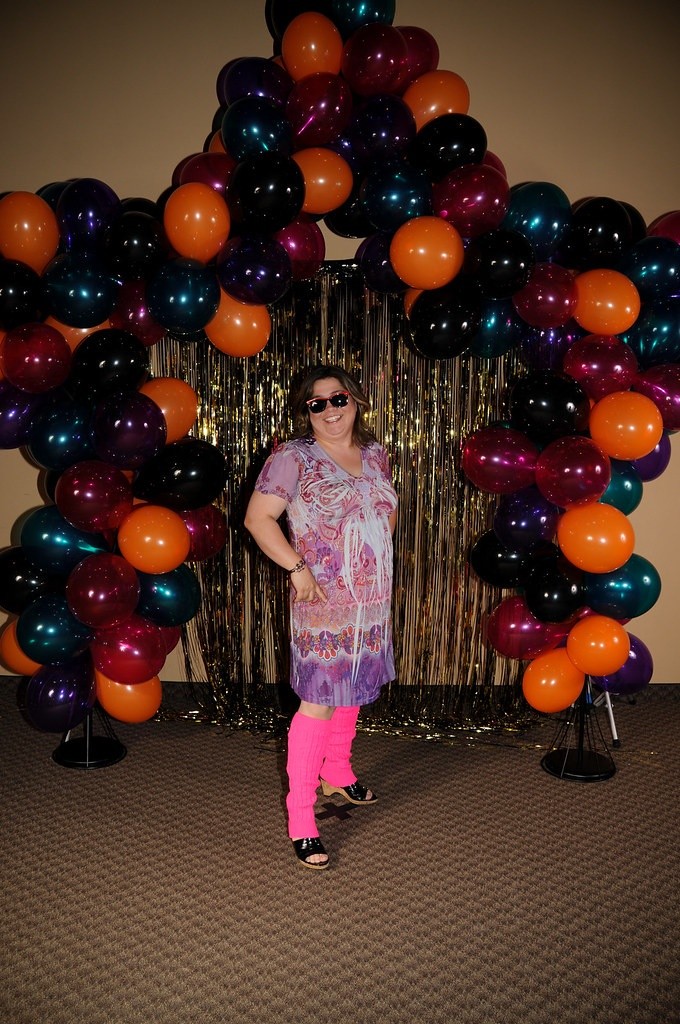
[245,365,398,867]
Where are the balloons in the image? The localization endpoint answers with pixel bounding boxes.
[570,269,640,334]
[293,148,353,213]
[118,506,190,574]
[588,391,663,460]
[566,615,630,677]
[1,0,679,733]
[141,378,198,442]
[204,288,271,358]
[94,670,162,724]
[164,183,230,263]
[557,502,635,573]
[521,648,585,712]
[390,216,464,291]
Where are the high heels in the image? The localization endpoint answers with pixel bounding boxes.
[292,837,329,870]
[320,778,377,804]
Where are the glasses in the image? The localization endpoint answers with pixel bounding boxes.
[307,391,349,413]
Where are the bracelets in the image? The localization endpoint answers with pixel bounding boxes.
[289,559,305,573]
